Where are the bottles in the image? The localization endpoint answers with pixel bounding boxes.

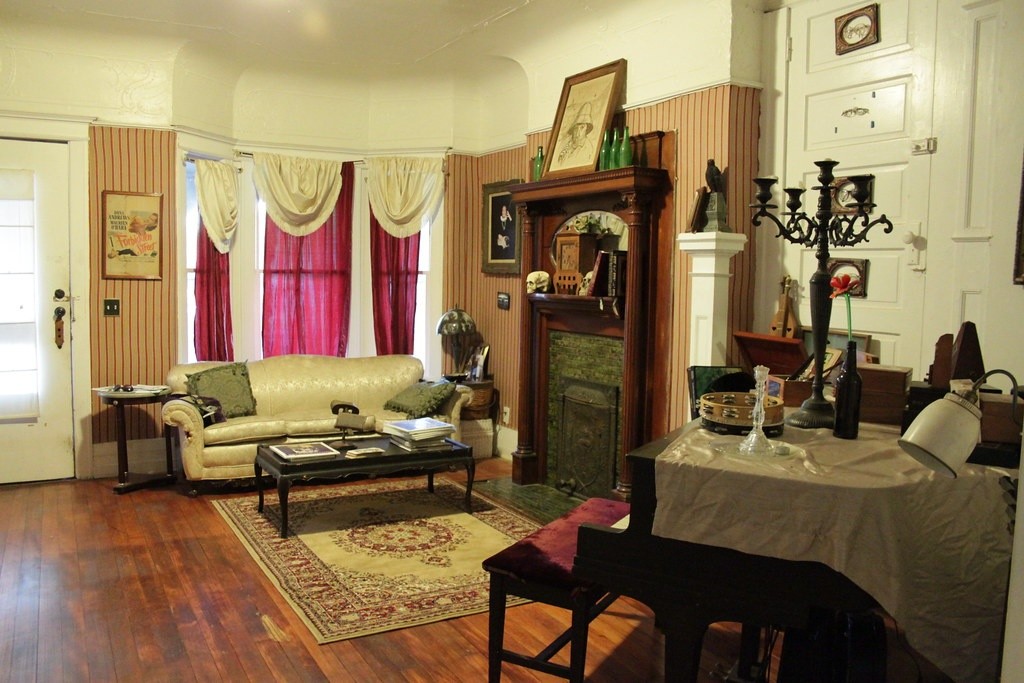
[610,127,620,168]
[599,129,611,171]
[619,126,632,167]
[534,146,544,181]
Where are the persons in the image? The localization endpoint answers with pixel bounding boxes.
[108,248,137,258]
[556,103,593,164]
[146,212,158,230]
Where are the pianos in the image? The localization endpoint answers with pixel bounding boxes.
[570,406,1019,683]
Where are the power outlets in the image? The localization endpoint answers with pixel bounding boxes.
[503,406,510,425]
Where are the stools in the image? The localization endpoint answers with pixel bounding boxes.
[481,497,661,683]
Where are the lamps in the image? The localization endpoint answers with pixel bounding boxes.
[435,302,476,382]
[896,369,1024,479]
[748,157,892,429]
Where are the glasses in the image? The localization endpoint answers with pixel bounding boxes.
[114,385,133,391]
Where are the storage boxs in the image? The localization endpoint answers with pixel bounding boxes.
[855,362,1024,469]
[765,347,843,407]
[460,378,496,420]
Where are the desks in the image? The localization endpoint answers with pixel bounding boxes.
[254,436,476,538]
[101,393,178,494]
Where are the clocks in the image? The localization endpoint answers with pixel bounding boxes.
[834,3,878,55]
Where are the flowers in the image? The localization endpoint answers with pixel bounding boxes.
[829,274,860,340]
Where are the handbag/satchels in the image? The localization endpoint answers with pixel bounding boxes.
[162,394,226,427]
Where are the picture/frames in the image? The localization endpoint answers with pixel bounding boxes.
[831,173,872,214]
[497,292,510,310]
[826,257,869,297]
[102,189,164,281]
[480,179,525,275]
[539,57,627,182]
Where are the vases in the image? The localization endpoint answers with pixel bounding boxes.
[833,340,863,439]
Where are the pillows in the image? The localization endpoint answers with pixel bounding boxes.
[184,362,257,418]
[383,376,457,420]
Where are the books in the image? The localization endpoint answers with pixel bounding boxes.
[608,249,628,297]
[382,416,456,452]
[269,441,340,463]
[587,251,609,295]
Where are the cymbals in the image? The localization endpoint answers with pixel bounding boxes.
[695,392,785,438]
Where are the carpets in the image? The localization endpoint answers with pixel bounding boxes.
[209,475,544,645]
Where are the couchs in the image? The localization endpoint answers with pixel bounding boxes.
[161,354,475,497]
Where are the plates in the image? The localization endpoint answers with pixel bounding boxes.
[442,374,468,383]
